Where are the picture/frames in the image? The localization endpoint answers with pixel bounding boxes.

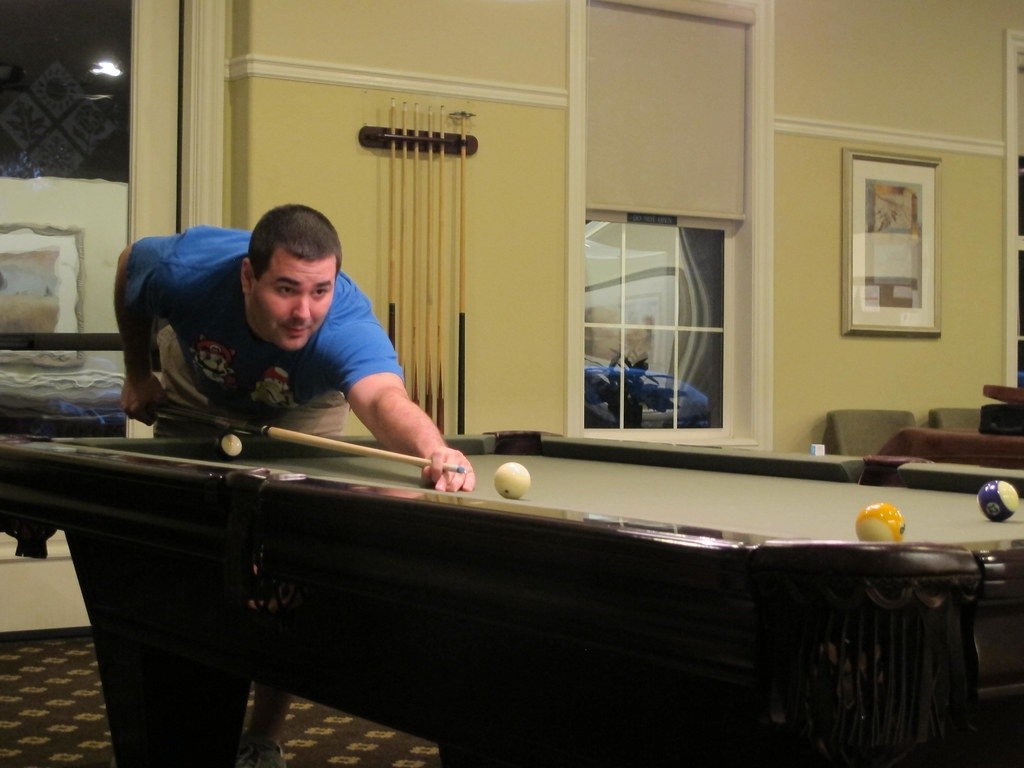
[0,223,86,367]
[839,146,943,337]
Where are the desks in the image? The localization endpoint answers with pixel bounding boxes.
[877,427,1024,469]
[0,430,1024,768]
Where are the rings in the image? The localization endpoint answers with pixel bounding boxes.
[464,470,466,474]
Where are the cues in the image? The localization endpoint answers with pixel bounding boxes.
[152,404,466,473]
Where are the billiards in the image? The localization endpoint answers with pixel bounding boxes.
[854,502,906,542]
[493,461,531,500]
[975,479,1020,522]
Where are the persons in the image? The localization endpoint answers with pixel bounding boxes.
[115,205,475,768]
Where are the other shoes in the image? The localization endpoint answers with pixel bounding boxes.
[236,735,286,768]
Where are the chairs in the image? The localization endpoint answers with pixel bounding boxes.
[828,409,917,455]
[930,408,981,429]
